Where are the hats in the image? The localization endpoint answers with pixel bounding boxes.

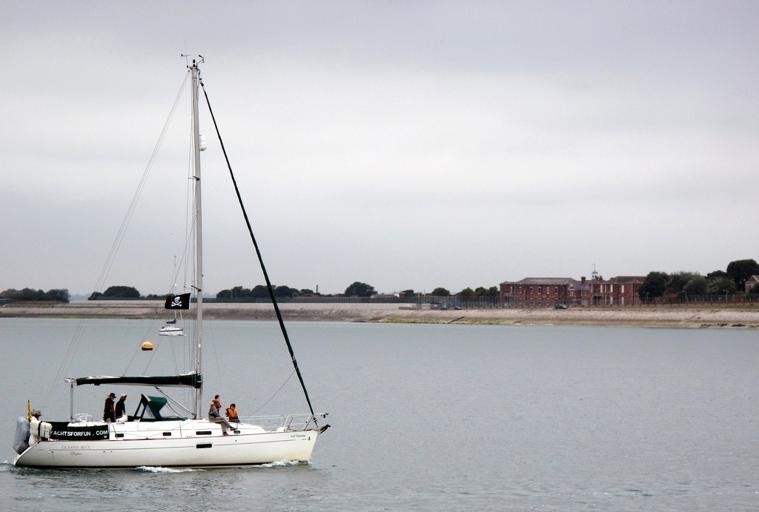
[107,393,116,398]
[120,393,127,397]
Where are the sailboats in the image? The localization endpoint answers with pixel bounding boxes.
[14,53,332,468]
[159,255,184,337]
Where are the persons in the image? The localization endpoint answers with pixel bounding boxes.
[115,393,127,423]
[103,393,116,423]
[211,394,222,414]
[225,403,240,423]
[208,402,238,436]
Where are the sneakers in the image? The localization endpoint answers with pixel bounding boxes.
[231,427,237,431]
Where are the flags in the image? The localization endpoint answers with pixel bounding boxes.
[164,293,190,310]
